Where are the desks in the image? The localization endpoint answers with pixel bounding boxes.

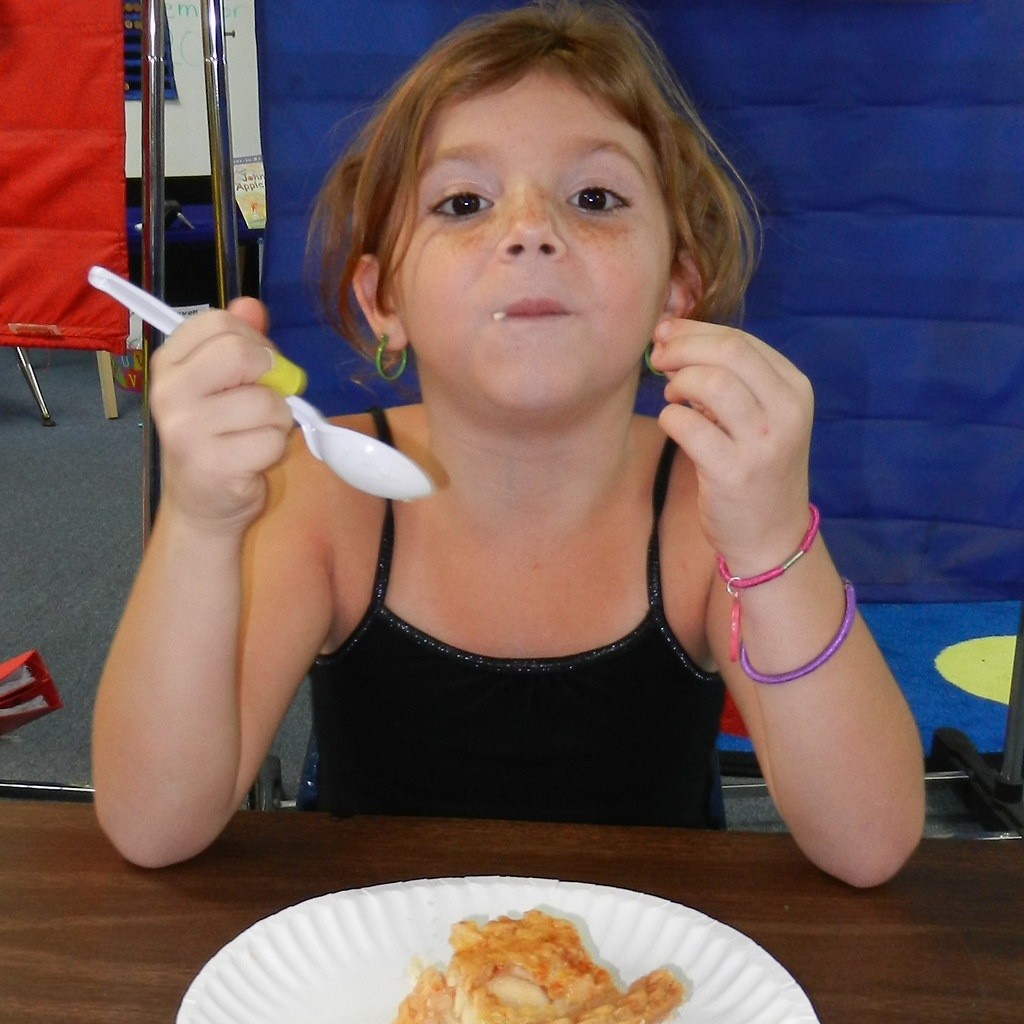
[0,795,1024,1024]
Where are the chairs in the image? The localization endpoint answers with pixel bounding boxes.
[198,0,1024,840]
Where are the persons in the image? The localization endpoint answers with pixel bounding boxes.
[90,0,925,886]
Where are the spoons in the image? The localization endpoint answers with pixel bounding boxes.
[86,265,432,504]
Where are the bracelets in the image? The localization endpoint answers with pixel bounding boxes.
[714,502,819,662]
[739,577,856,683]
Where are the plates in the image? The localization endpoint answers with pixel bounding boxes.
[174,876,823,1024]
